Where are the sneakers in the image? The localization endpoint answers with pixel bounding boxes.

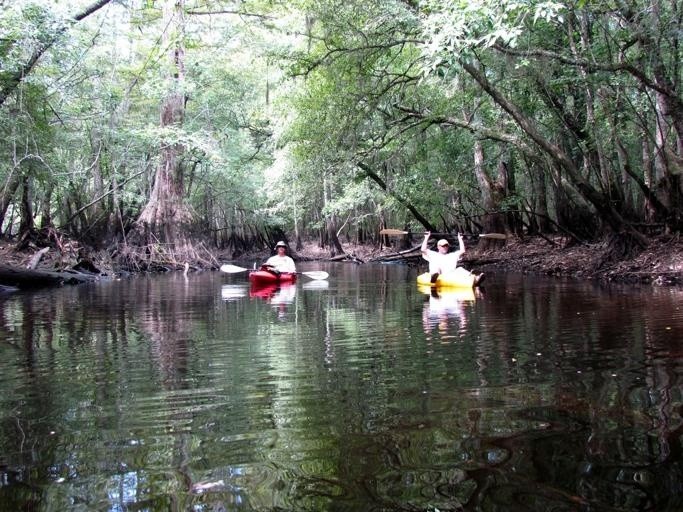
[431,273,438,283]
[474,273,484,285]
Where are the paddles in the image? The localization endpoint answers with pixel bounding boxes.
[380,229,506,239]
[221,265,329,279]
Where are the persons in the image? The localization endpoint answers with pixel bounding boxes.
[420,231,486,286]
[265,241,297,275]
[268,282,297,320]
[421,295,469,342]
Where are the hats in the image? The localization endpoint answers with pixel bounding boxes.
[437,239,449,246]
[274,241,288,250]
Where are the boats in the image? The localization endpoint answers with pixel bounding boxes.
[249,266,296,283]
[416,272,475,289]
